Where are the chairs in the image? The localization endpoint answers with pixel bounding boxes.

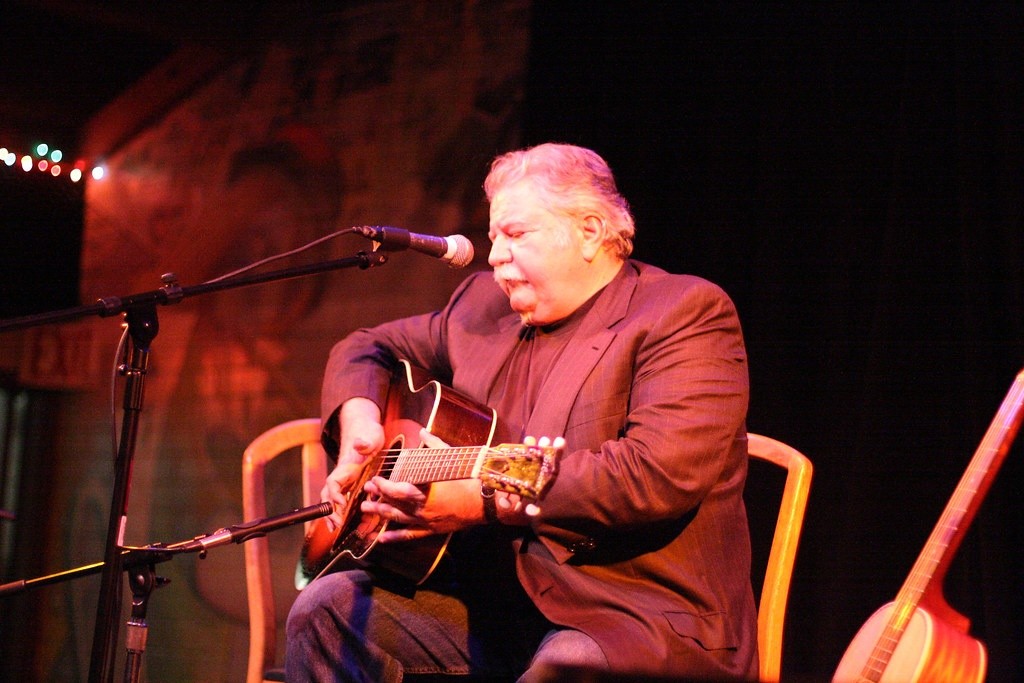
[747,430,814,683]
[243,419,331,681]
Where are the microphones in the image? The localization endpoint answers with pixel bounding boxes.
[352,226,475,270]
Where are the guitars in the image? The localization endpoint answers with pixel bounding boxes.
[830,368,1024,683]
[294,359,566,590]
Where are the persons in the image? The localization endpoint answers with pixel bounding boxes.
[285,143,762,683]
